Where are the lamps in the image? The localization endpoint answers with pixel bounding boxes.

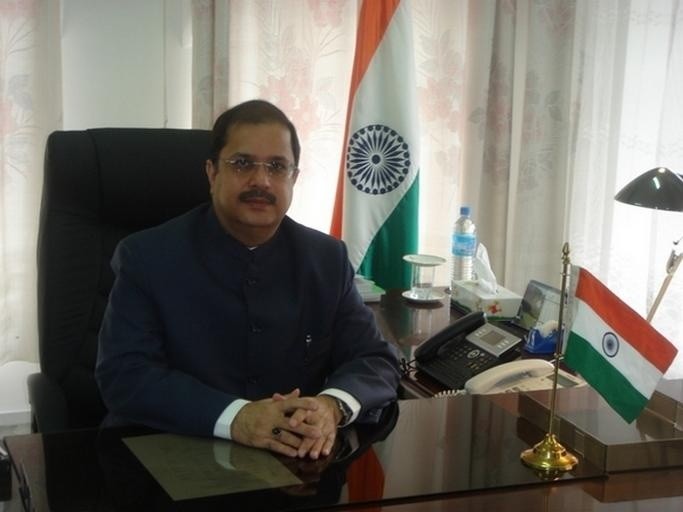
[614,168,683,322]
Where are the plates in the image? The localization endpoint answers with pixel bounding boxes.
[402,253,447,267]
[401,289,447,304]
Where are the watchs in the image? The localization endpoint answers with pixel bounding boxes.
[330,397,353,426]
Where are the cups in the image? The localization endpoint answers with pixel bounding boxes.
[412,264,435,299]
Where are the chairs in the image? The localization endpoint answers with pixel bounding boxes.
[26,128,253,430]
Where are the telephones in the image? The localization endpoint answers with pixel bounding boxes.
[430,359,587,399]
[399,310,526,392]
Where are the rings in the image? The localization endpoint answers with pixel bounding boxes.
[272,427,282,435]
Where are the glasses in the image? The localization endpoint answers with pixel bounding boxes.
[220,156,299,180]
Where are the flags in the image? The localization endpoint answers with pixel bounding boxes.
[561,263,679,426]
[330,1,420,291]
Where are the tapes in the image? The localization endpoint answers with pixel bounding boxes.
[533,320,564,338]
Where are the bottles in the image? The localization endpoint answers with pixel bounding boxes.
[450,207,477,291]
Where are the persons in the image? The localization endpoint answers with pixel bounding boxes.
[95,99,400,460]
